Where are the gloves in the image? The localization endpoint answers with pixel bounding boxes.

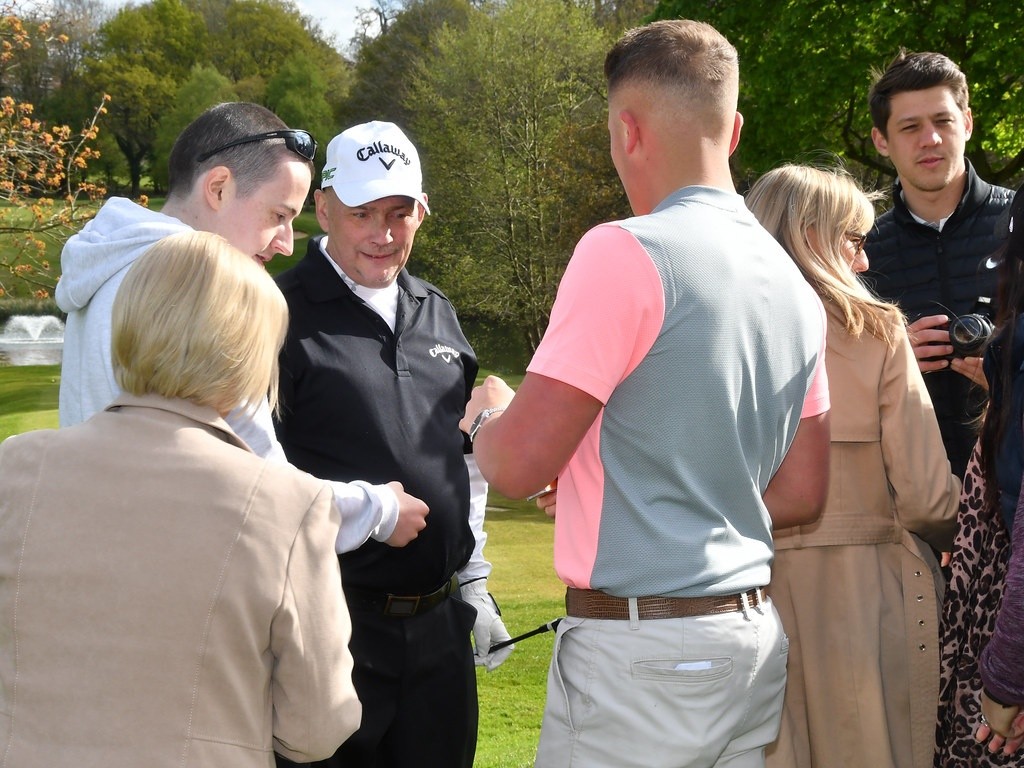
[458,574,516,672]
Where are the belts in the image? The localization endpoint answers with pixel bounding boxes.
[354,570,460,619]
[565,585,766,621]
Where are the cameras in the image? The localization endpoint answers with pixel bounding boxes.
[926,296,997,370]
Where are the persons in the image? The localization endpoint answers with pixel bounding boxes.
[55,103,430,556]
[271,118,514,768]
[0,231,363,768]
[459,19,832,768]
[746,53,1024,768]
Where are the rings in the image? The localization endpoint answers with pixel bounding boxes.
[980,716,987,725]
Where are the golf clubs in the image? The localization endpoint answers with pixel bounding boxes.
[487,615,566,653]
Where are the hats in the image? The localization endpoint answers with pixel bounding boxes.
[321,121,431,217]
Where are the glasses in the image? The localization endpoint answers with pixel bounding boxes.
[845,229,868,253]
[197,128,318,161]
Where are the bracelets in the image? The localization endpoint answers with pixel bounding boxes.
[983,684,1013,708]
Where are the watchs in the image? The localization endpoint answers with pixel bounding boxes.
[468,403,504,443]
